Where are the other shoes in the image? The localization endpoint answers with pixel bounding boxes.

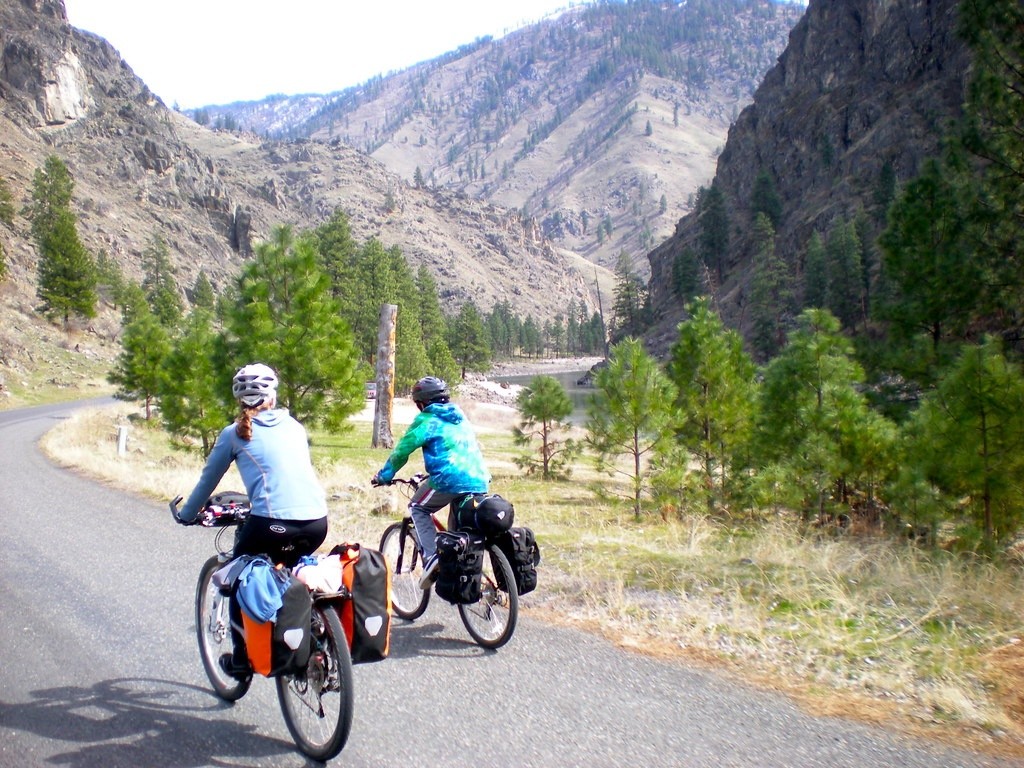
[218,653,253,683]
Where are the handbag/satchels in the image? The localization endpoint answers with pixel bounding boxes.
[489,526,540,594]
[329,543,391,664]
[228,559,309,679]
[435,528,483,605]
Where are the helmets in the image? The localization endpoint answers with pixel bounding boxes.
[412,377,450,403]
[232,364,279,398]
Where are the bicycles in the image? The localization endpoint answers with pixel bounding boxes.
[169,489,355,761]
[369,473,519,649]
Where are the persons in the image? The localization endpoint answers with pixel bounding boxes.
[175,363,329,683]
[372,377,489,587]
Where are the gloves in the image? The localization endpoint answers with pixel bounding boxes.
[177,511,200,526]
[374,473,391,486]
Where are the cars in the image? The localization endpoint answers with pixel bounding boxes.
[366,382,376,399]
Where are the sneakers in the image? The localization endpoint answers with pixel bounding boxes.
[418,553,439,590]
